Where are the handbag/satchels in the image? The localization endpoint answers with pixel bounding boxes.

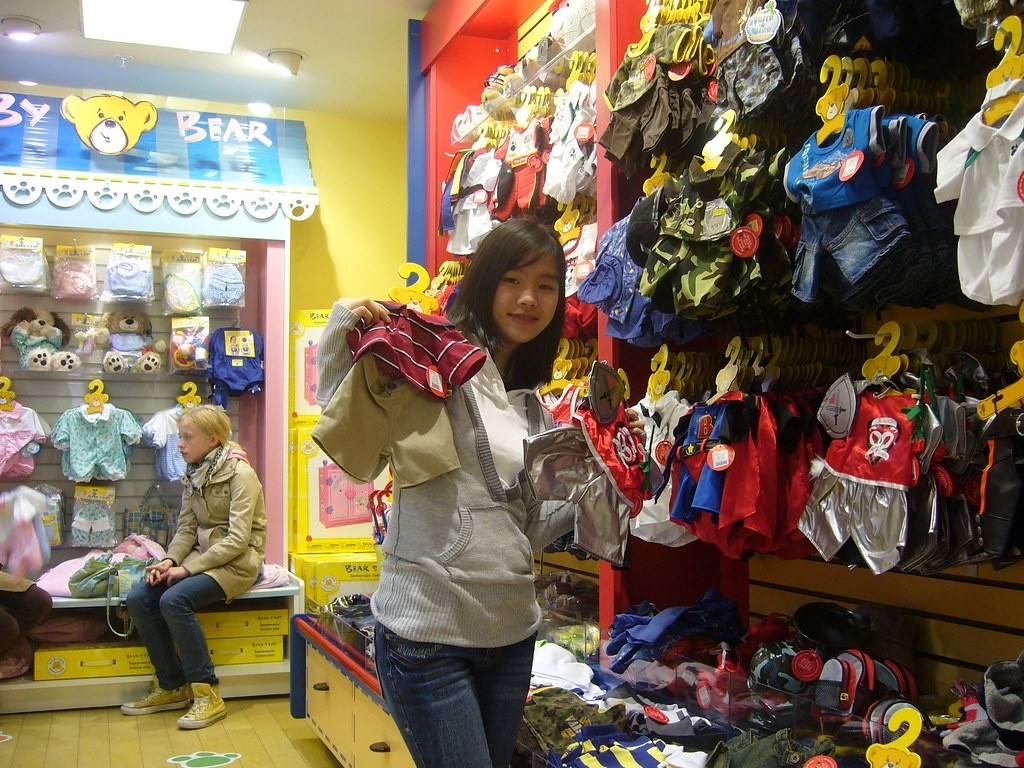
[811,648,933,745]
[68,549,160,637]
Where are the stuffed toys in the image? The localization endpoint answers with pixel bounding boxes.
[453,0,597,142]
[1,306,81,371]
[109,537,152,564]
[102,310,167,374]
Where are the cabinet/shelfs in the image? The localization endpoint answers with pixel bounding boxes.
[0,562,305,713]
[288,612,415,767]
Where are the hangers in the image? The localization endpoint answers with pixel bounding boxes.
[0,377,16,412]
[83,379,109,415]
[474,0,1024,246]
[230,318,243,329]
[176,382,202,414]
[388,257,1003,407]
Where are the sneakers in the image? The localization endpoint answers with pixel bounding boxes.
[177,682,227,729]
[121,674,190,716]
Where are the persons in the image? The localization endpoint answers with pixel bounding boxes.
[121,405,266,728]
[317,221,647,768]
[0,564,52,657]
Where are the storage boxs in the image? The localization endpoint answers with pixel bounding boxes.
[33,608,289,681]
[517,0,565,59]
[294,545,384,608]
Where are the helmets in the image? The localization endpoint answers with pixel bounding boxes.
[747,641,807,695]
[793,602,859,664]
